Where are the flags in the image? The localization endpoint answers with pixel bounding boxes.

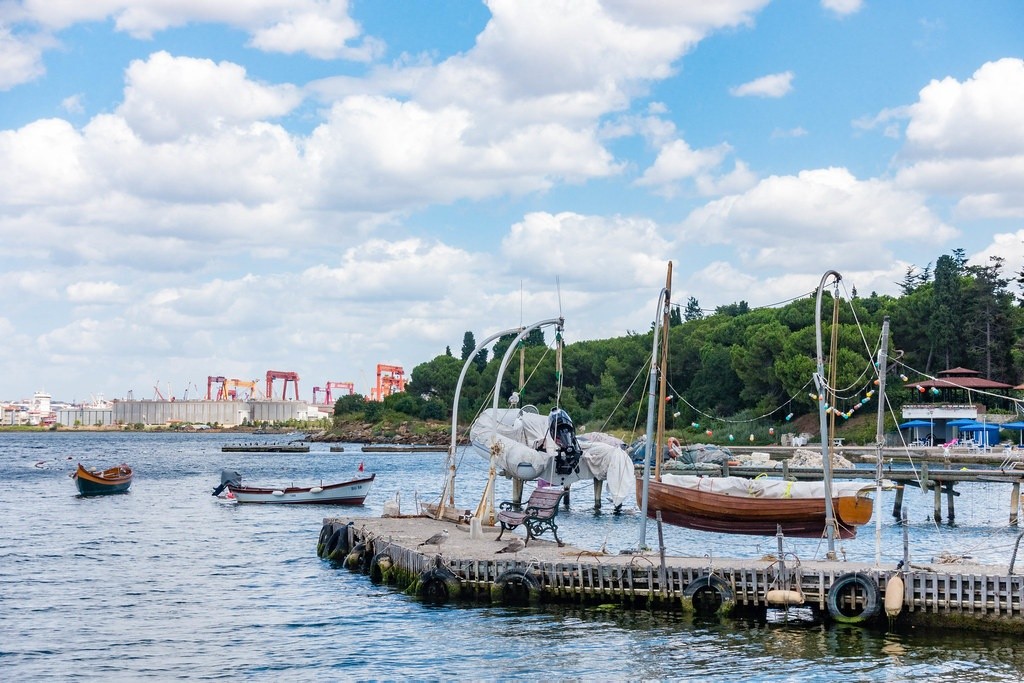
[359,462,363,472]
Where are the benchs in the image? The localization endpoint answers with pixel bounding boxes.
[494,487,569,548]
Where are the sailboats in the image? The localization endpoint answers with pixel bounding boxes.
[634,261,903,539]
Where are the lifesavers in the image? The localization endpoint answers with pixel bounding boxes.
[666,435,683,459]
[680,575,738,619]
[316,524,333,558]
[368,553,395,585]
[489,567,542,605]
[826,570,881,626]
[413,568,462,604]
[322,528,348,561]
[348,543,373,574]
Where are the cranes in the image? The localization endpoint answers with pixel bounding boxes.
[86,380,280,408]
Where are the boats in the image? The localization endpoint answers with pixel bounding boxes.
[72,462,134,497]
[221,442,311,453]
[361,443,449,452]
[330,443,344,452]
[212,469,376,506]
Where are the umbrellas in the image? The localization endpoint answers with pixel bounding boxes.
[1000,422,1024,446]
[899,420,935,445]
[947,419,998,440]
